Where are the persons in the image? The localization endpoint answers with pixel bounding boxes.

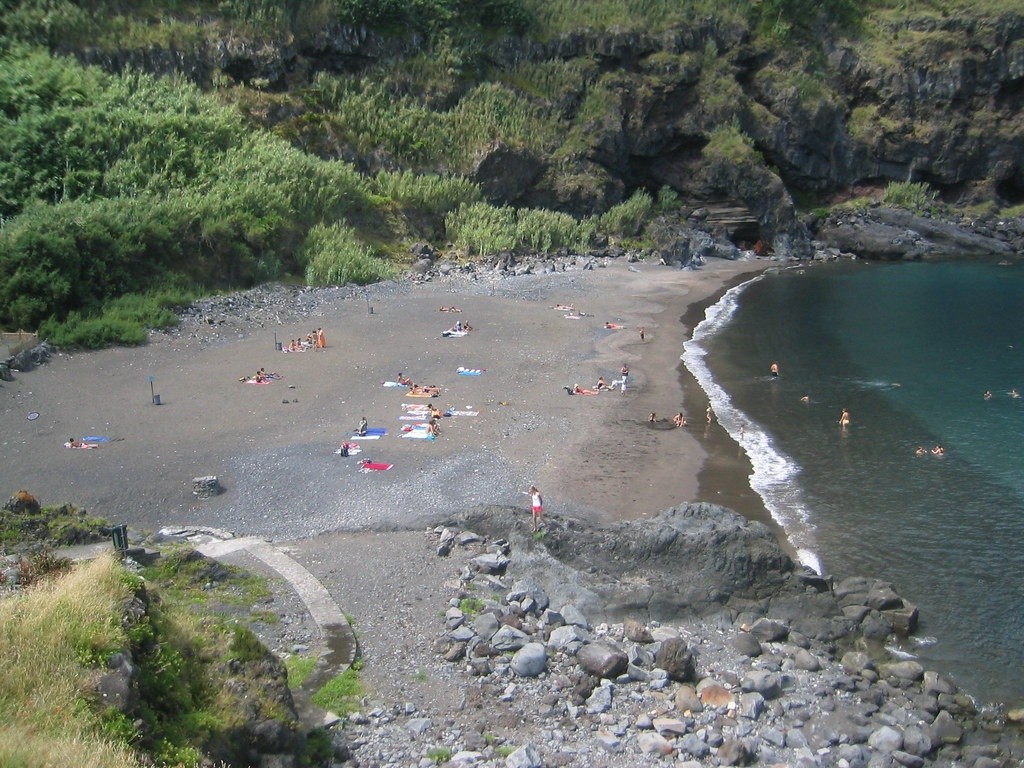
[254,327,368,458]
[840,408,849,430]
[529,486,547,532]
[605,321,627,330]
[801,394,809,402]
[739,424,746,441]
[986,391,990,395]
[439,306,463,313]
[639,327,645,342]
[916,447,926,453]
[648,412,657,423]
[931,445,943,453]
[443,319,473,334]
[69,438,98,450]
[771,361,779,377]
[673,412,686,428]
[397,372,442,437]
[557,304,575,310]
[1012,389,1017,394]
[565,363,629,396]
[705,403,712,423]
